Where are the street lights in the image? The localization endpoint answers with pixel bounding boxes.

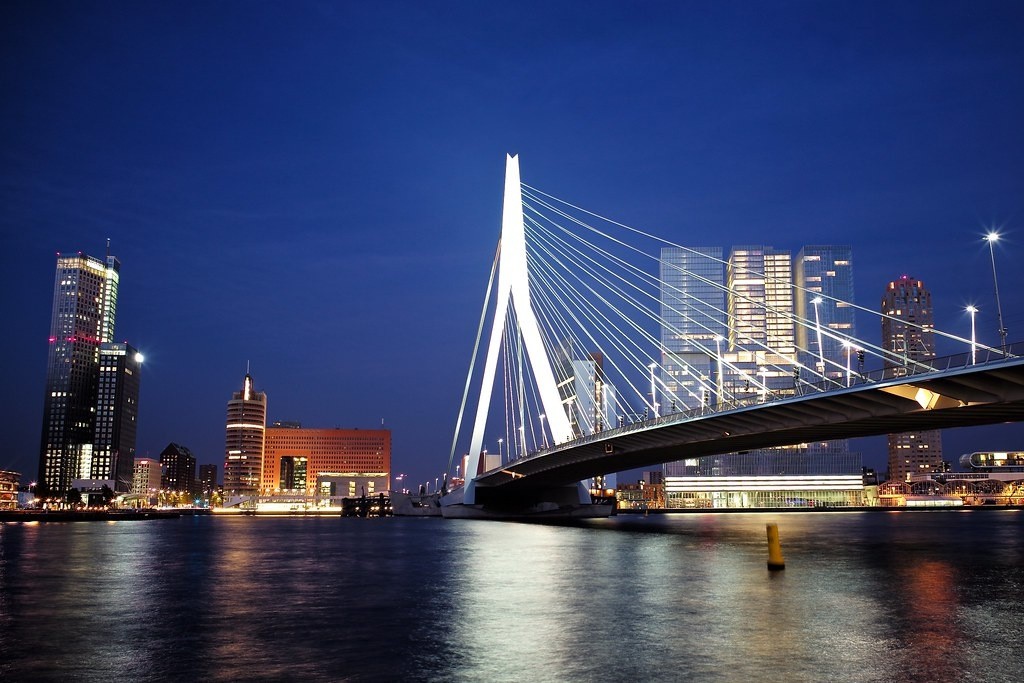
[843,341,850,388]
[648,363,660,425]
[483,450,487,472]
[988,232,1006,359]
[813,297,827,392]
[699,387,705,415]
[427,482,429,494]
[539,414,545,451]
[760,366,765,402]
[400,474,407,489]
[497,439,503,466]
[444,474,447,480]
[436,478,438,490]
[566,401,573,440]
[419,485,422,493]
[967,306,975,365]
[601,384,607,420]
[456,465,460,485]
[518,427,523,455]
[714,336,723,402]
